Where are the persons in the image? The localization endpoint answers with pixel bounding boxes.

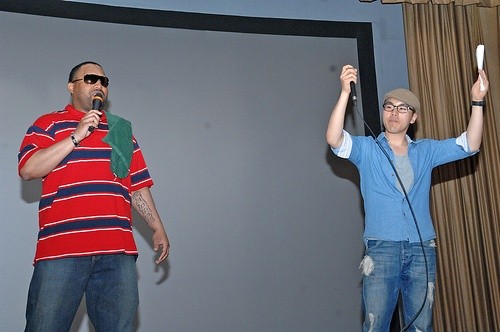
[18,61,170,332]
[326,64,489,332]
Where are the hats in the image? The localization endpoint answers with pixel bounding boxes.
[384,88,420,114]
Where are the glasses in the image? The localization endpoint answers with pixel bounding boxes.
[72,74,109,88]
[382,102,415,113]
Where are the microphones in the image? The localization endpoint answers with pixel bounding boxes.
[350,81,357,104]
[88,92,103,132]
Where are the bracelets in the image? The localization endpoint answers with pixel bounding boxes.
[471,100,485,106]
[70,131,79,146]
